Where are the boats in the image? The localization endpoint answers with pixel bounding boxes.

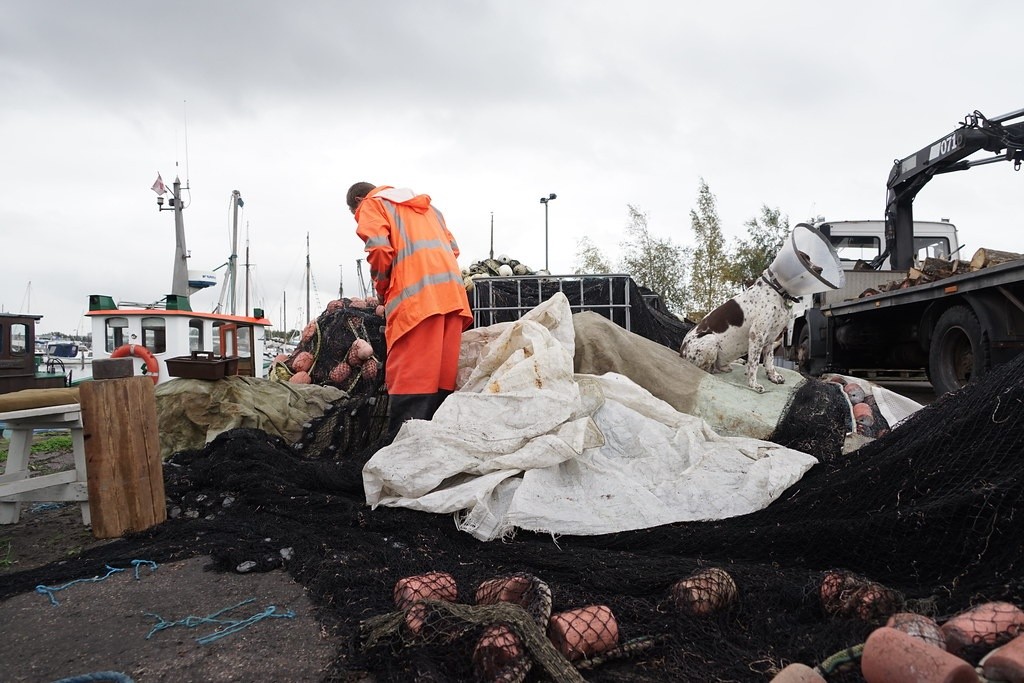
[1,101,312,392]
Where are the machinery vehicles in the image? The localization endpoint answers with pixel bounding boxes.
[782,107,1024,401]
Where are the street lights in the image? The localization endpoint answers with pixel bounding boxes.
[540,193,558,270]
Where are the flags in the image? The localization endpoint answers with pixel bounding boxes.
[151,174,166,196]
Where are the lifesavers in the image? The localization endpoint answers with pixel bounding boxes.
[110,343,160,385]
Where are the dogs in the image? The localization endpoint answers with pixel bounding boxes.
[678,249,824,394]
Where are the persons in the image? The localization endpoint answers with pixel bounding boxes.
[346,181,474,448]
